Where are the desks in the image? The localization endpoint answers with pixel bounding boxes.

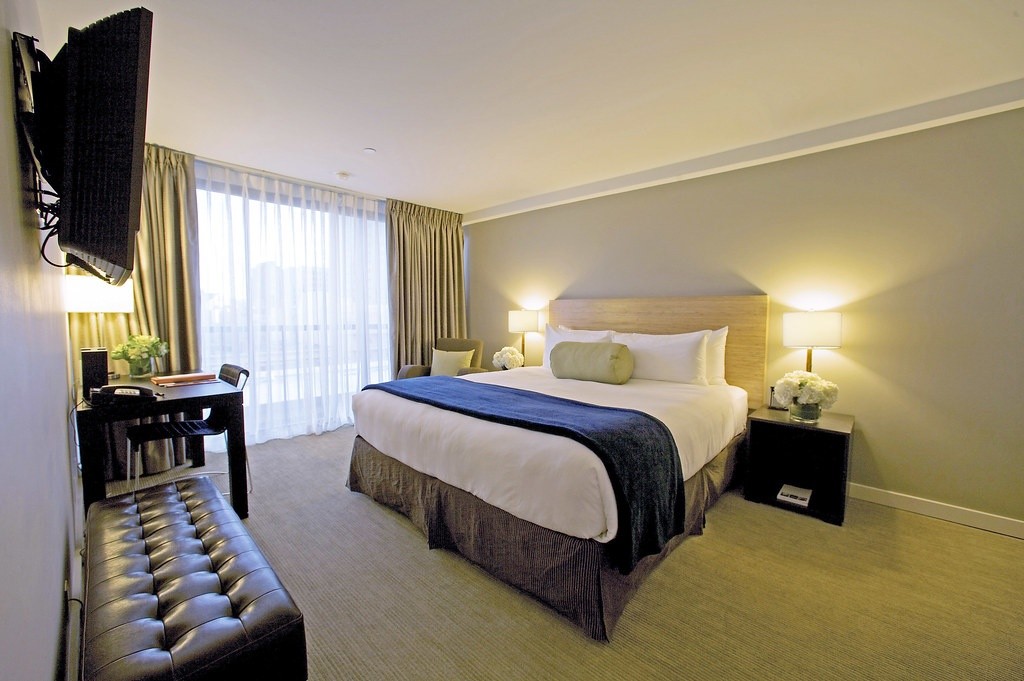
[76,370,248,520]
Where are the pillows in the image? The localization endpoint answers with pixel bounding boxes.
[430,347,475,376]
[543,323,728,387]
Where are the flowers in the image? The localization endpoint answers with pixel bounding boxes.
[774,370,839,409]
[111,334,169,374]
[492,346,525,370]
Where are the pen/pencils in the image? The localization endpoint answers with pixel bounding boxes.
[155,392,165,398]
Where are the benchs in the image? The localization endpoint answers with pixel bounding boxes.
[80,474,308,681]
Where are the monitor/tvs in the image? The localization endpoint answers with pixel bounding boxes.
[22,6,154,287]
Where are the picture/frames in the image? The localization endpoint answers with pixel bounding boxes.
[769,387,788,411]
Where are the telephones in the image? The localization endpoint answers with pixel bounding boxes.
[90,385,157,404]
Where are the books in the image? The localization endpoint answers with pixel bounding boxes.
[150,373,221,388]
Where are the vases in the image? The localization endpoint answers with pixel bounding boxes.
[790,403,821,424]
[129,359,152,377]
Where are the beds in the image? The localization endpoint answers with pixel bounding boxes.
[346,295,769,643]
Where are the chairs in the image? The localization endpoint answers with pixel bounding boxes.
[127,364,253,495]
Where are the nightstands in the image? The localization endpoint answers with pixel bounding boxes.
[744,403,854,526]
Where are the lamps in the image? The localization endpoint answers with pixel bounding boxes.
[783,312,841,372]
[66,274,134,380]
[508,309,538,367]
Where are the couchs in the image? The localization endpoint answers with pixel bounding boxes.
[398,338,488,380]
